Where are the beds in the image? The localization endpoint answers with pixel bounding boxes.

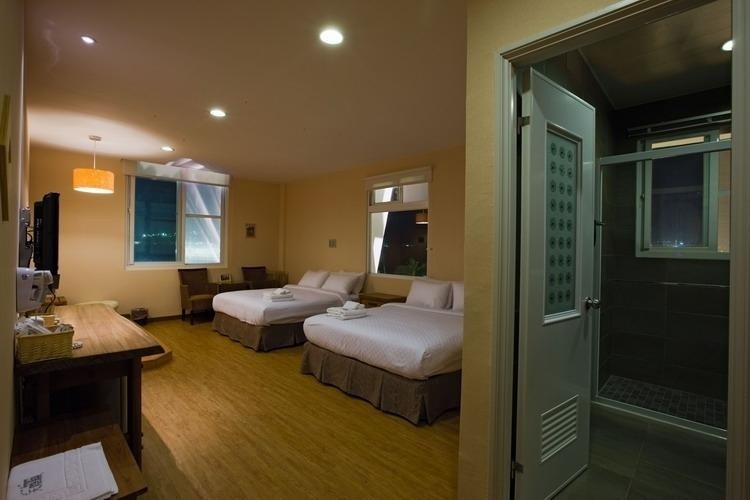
[299,275,464,426]
[212,268,367,352]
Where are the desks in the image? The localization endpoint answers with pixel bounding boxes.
[17,300,165,475]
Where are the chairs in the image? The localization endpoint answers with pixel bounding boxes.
[241,266,277,290]
[177,267,219,325]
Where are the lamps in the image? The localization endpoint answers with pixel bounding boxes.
[416,209,428,224]
[72,135,115,195]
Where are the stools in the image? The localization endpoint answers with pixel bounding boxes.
[8,424,149,500]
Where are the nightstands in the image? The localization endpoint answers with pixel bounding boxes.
[358,292,406,308]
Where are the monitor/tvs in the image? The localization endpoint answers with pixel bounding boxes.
[33,192,61,291]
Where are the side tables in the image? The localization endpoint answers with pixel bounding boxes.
[216,282,246,294]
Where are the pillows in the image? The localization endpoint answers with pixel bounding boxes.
[340,270,366,295]
[452,283,464,313]
[405,278,452,310]
[321,273,359,295]
[298,270,328,289]
